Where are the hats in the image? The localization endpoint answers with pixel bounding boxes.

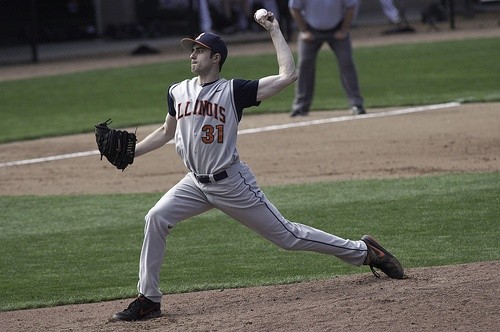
[181,31,228,60]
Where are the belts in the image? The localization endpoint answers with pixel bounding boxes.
[193,170,229,184]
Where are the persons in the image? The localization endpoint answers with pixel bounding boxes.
[95,8,404,322]
[290,0,367,118]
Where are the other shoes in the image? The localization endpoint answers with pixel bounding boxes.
[351,105,364,114]
[290,110,308,118]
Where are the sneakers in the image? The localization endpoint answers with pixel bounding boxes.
[113,293,161,322]
[361,235,406,279]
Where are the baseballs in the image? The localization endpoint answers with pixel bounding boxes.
[256,8,269,22]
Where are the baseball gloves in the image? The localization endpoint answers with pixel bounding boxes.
[93,121,137,170]
